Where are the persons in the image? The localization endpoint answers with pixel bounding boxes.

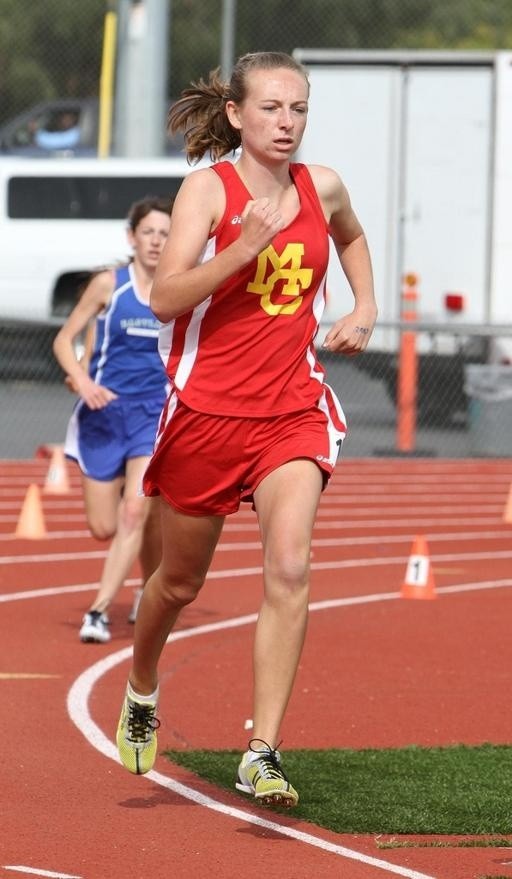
[111,46,380,814]
[61,315,165,626]
[51,189,183,648]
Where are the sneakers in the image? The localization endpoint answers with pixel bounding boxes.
[117,679,160,774]
[80,609,112,641]
[234,737,299,809]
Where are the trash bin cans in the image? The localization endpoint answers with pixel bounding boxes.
[462,363,512,459]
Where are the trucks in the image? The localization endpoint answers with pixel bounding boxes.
[2,97,189,156]
[292,49,512,425]
